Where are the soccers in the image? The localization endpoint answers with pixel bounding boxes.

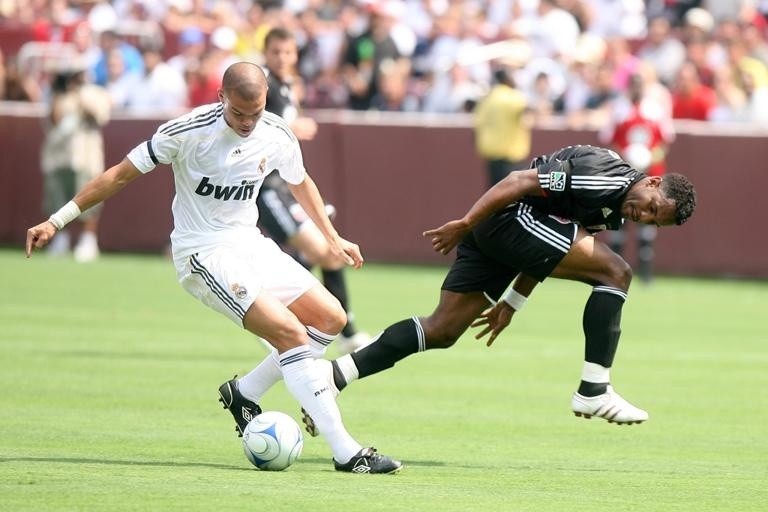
[242,411,302,470]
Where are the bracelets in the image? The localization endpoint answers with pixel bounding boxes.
[47,200,83,233]
[501,286,530,313]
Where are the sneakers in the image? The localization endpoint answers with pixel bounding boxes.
[568,384,650,425]
[216,375,264,439]
[301,357,343,437]
[330,445,405,475]
[338,328,374,353]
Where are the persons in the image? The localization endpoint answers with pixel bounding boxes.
[31,44,112,260]
[298,143,696,437]
[22,60,408,476]
[247,29,373,351]
[0,0,768,127]
[595,72,675,286]
[470,68,530,197]
